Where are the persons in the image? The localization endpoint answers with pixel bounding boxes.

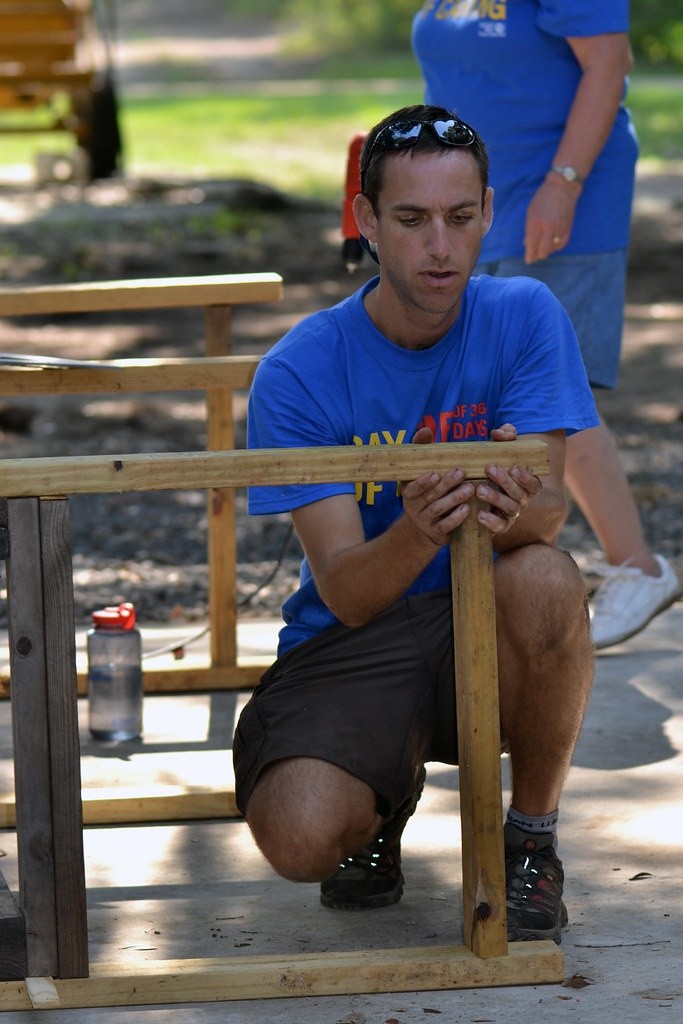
[231,105,601,941]
[410,0,683,648]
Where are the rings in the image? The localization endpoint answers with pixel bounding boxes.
[502,511,519,519]
[532,474,543,496]
[552,237,561,244]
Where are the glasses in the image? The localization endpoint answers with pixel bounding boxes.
[360,120,483,196]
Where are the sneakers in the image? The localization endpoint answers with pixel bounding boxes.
[505,836,568,944]
[589,553,681,649]
[321,765,430,911]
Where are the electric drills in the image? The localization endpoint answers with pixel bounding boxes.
[341,136,373,276]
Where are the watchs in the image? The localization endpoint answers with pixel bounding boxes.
[550,164,586,187]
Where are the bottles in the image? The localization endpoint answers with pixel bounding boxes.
[87,602,143,741]
